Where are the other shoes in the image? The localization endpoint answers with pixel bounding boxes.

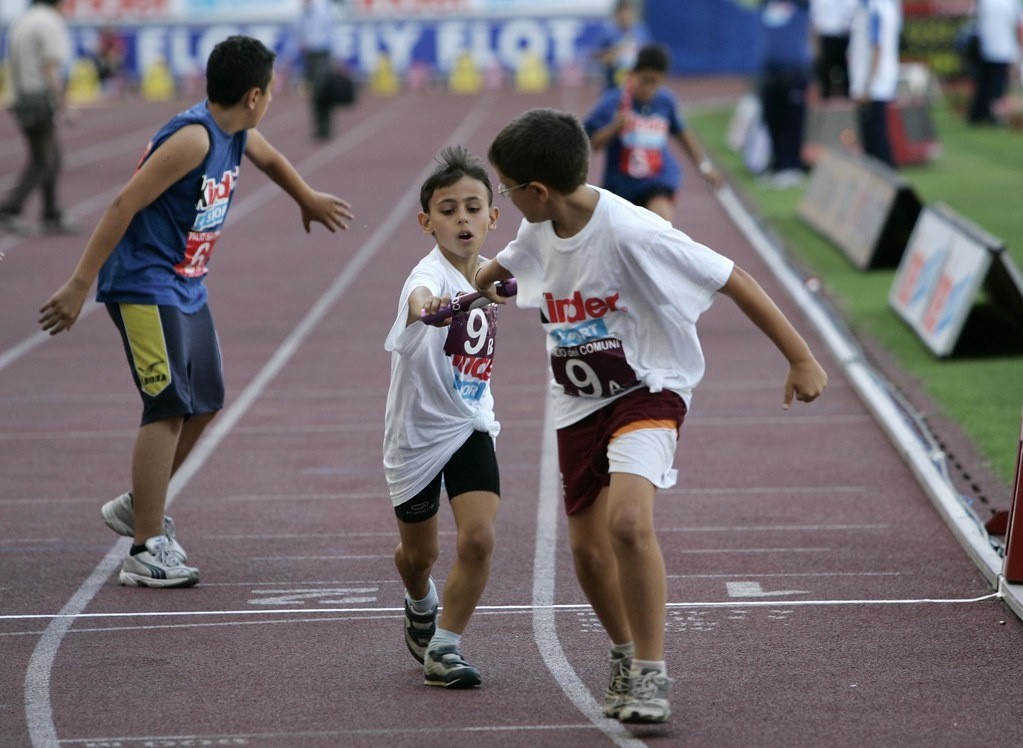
[42,218,83,236]
[1,211,24,234]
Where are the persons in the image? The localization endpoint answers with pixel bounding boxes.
[0,1,73,231]
[845,1,901,165]
[474,106,829,724]
[39,35,353,586]
[380,148,501,687]
[592,1,650,91]
[739,0,812,173]
[293,0,344,140]
[583,44,723,216]
[810,1,860,97]
[965,0,1022,126]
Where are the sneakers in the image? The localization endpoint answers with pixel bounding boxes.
[620,664,675,721]
[403,598,439,664]
[100,493,175,541]
[422,643,481,689]
[120,536,199,589]
[604,650,633,717]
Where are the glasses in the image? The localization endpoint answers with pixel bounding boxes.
[498,183,530,198]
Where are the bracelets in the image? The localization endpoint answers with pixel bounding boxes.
[474,265,486,292]
